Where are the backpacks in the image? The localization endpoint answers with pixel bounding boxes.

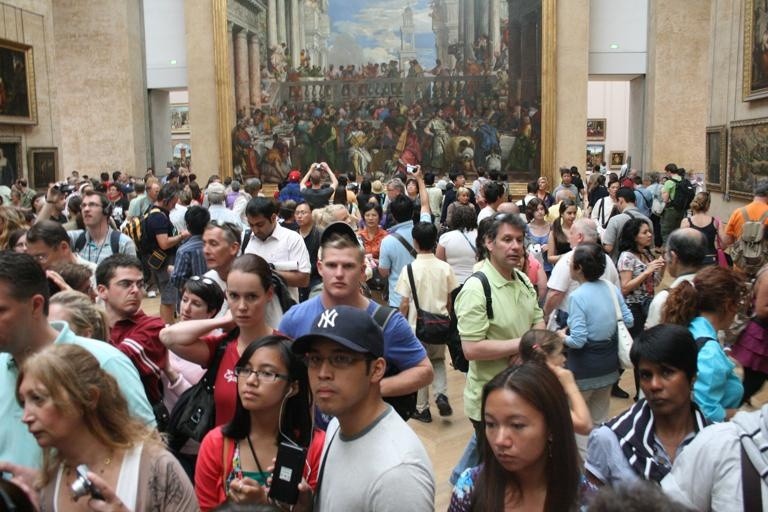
[443,272,529,374]
[731,205,768,275]
[120,208,166,251]
[668,177,694,212]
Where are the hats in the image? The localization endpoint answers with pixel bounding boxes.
[292,306,384,356]
[290,170,301,183]
[207,181,224,194]
[321,222,361,247]
[371,180,384,195]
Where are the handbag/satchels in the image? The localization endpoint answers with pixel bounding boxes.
[411,311,454,345]
[617,321,634,369]
[168,381,217,444]
[153,400,191,454]
[728,291,757,336]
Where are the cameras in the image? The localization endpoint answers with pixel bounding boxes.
[52,183,73,192]
[71,464,101,500]
[407,165,416,172]
[316,164,323,169]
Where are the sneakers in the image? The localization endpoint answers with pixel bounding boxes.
[410,407,432,424]
[435,392,453,416]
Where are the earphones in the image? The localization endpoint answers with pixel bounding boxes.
[286,386,297,397]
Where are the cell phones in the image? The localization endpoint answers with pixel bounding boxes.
[269,443,305,504]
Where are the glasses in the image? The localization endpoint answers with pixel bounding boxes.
[306,349,365,369]
[188,274,213,286]
[234,366,293,385]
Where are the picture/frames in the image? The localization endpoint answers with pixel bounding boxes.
[705,122,728,194]
[28,144,59,192]
[586,117,608,141]
[741,0,768,103]
[586,143,606,176]
[608,149,626,170]
[0,37,39,127]
[0,135,25,191]
[209,0,559,201]
[726,117,768,201]
[169,102,191,134]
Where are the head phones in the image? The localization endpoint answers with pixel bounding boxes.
[163,187,176,205]
[104,203,113,216]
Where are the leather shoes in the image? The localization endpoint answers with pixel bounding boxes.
[613,388,627,397]
